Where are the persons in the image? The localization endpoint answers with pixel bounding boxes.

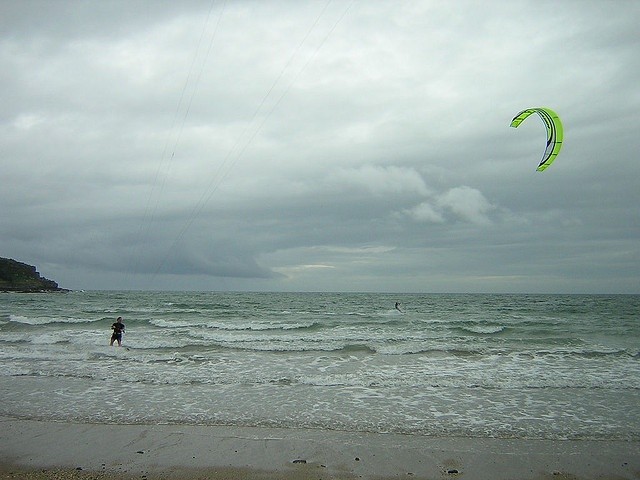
[394,302,403,313]
[108,316,127,347]
